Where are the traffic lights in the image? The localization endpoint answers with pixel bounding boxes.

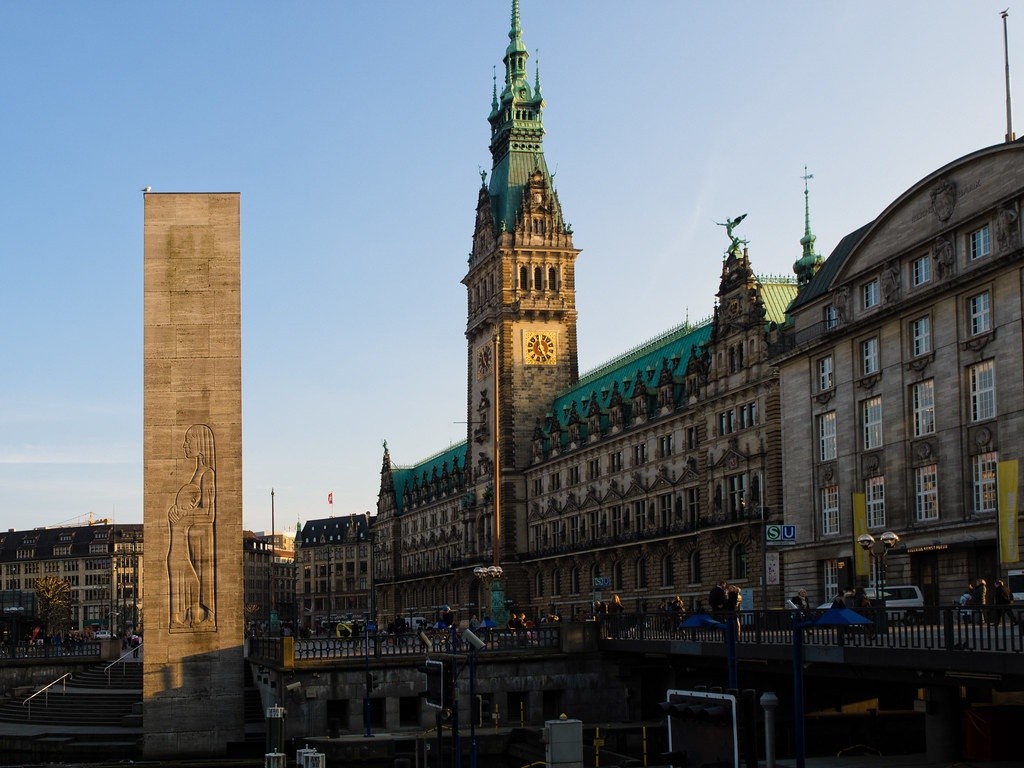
[658,686,755,731]
[419,659,444,708]
[475,695,490,729]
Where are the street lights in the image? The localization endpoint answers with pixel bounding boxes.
[4,606,26,659]
[859,531,900,617]
[472,565,503,637]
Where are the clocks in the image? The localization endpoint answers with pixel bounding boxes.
[475,336,493,383]
[522,329,562,368]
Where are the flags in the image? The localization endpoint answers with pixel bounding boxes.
[328,493,332,504]
[998,460,1019,562]
[853,493,870,575]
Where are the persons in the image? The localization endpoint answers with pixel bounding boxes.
[442,605,563,645]
[551,172,556,185]
[501,219,506,231]
[709,581,743,641]
[383,439,388,452]
[24,631,140,658]
[657,596,685,637]
[244,613,406,648]
[567,223,572,230]
[479,170,487,184]
[792,586,875,640]
[717,218,750,250]
[594,594,624,638]
[964,579,1018,625]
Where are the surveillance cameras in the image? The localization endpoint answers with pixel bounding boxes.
[417,631,432,648]
[463,629,486,651]
[286,682,301,690]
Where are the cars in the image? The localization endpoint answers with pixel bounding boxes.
[817,585,926,627]
[97,629,118,640]
